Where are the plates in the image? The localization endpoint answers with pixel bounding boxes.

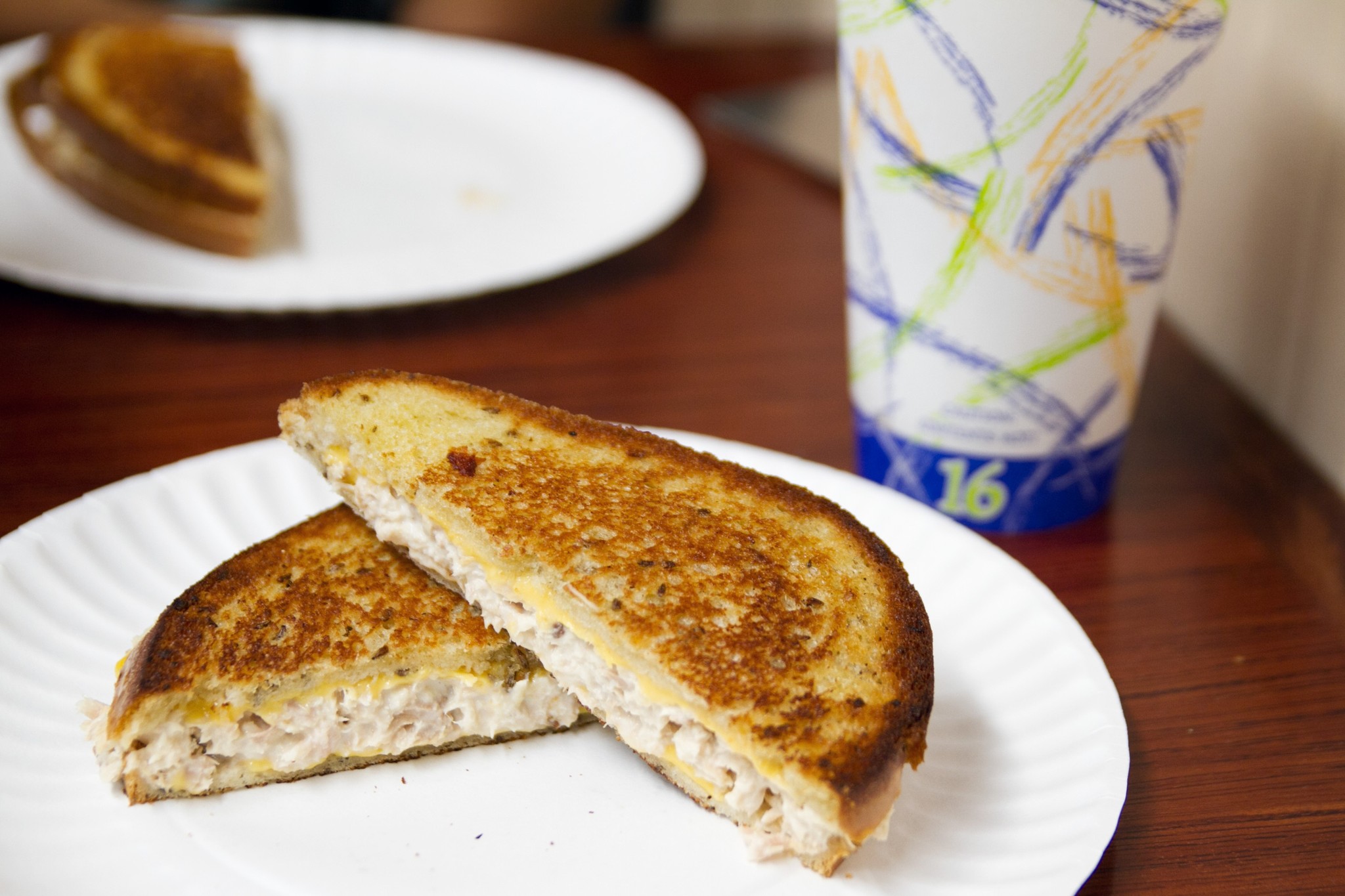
[0,420,1132,896]
[0,17,708,312]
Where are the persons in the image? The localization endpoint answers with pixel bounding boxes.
[0,0,667,72]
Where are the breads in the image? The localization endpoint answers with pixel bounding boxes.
[279,368,934,876]
[108,503,602,803]
[7,17,267,259]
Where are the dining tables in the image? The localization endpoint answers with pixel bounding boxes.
[0,27,1345,896]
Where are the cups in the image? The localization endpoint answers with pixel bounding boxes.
[835,1,1225,534]
